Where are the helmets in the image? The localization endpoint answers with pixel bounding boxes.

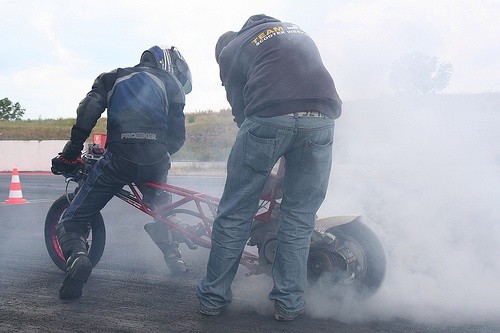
[140,44,193,94]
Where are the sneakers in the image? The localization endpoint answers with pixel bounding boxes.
[166,256,191,275]
[59,252,92,300]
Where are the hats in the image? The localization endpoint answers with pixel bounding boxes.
[215,32,238,62]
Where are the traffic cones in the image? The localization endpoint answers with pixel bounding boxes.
[4,168,28,205]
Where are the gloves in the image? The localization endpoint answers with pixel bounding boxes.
[60,141,84,161]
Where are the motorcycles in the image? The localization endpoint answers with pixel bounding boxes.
[43,143,386,304]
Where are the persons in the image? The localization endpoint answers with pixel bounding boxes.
[196,14,342,321]
[51,45,193,298]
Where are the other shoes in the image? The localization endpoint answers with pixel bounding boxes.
[274,309,305,321]
[199,305,227,316]
